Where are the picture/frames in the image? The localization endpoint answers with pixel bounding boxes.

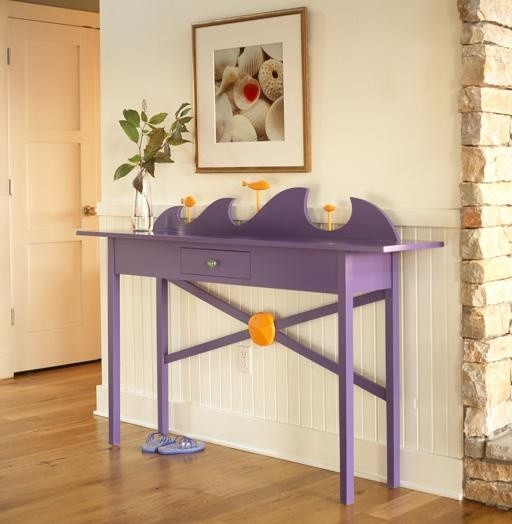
[76,188,445,507]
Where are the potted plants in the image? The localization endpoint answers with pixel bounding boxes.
[190,8,312,173]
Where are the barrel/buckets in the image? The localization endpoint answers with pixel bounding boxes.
[157,435,205,455]
[142,431,176,453]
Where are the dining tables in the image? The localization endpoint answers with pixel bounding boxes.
[113,99,195,232]
